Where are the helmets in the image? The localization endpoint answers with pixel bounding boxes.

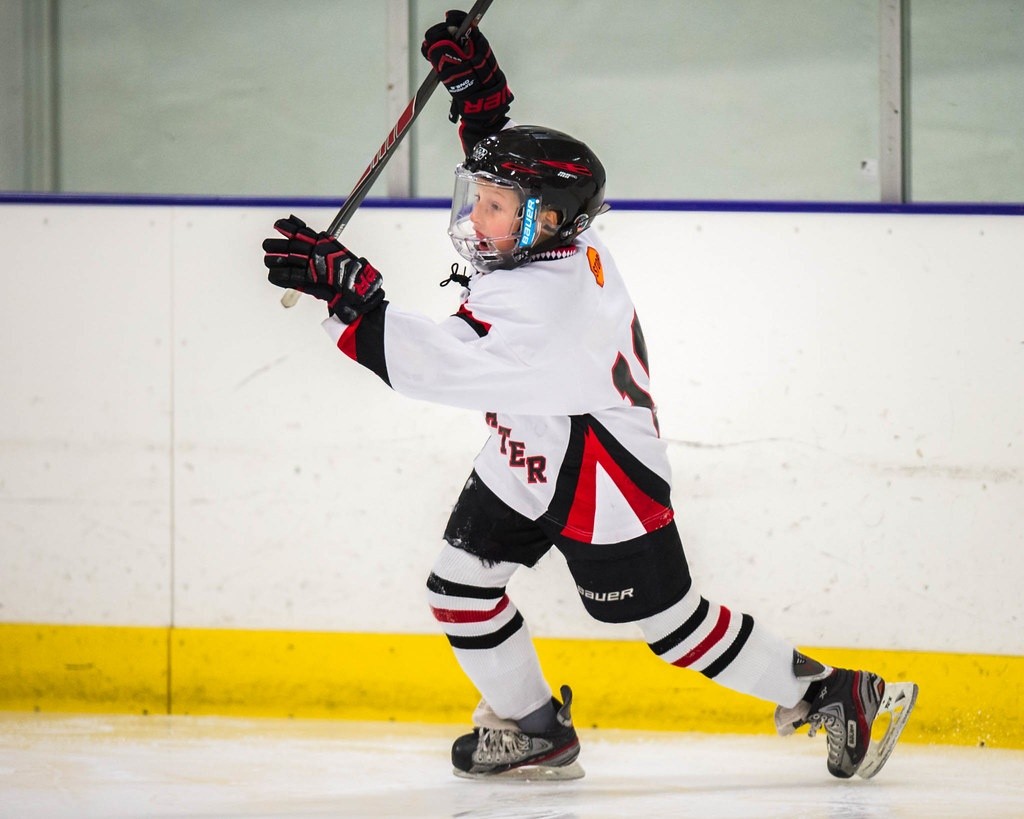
[461,125,607,248]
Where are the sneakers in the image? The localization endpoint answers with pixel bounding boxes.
[774,647,919,781]
[451,684,585,781]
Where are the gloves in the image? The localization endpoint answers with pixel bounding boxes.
[262,215,385,324]
[421,10,515,123]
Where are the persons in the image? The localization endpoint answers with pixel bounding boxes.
[263,9,920,779]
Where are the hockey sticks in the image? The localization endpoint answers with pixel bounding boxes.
[280,0,495,311]
